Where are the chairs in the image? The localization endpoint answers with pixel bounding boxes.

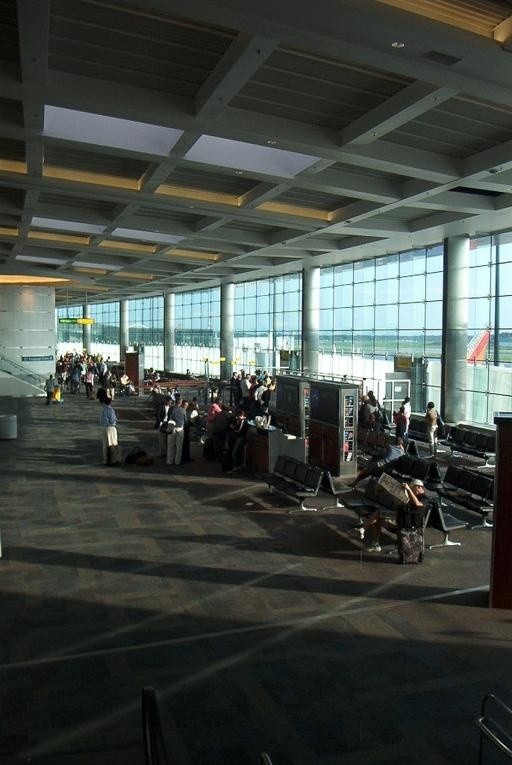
[253,402,496,564]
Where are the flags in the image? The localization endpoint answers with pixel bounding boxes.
[466,329,490,366]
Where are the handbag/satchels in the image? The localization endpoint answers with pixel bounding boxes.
[125,446,153,466]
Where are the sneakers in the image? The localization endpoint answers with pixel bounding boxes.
[350,528,365,539]
[364,544,381,552]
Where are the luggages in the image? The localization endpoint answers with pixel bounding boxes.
[398,506,425,564]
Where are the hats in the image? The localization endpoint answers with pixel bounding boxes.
[408,479,424,486]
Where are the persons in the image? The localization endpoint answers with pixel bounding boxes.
[44,373,58,408]
[343,478,430,553]
[143,366,276,476]
[347,435,407,488]
[357,388,413,440]
[99,397,122,467]
[55,350,139,401]
[423,401,441,451]
